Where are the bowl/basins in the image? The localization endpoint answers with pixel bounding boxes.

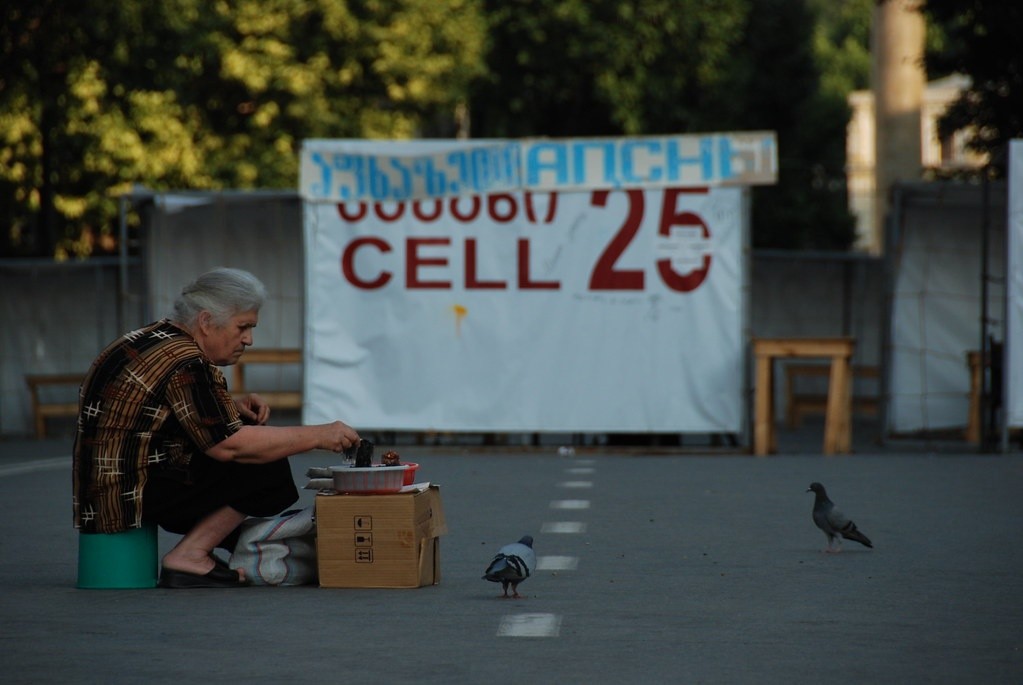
[327,464,409,495]
[375,462,419,485]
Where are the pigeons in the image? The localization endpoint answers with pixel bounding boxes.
[806,482,873,553]
[480,534,538,599]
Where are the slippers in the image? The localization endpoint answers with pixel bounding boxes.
[160,552,244,589]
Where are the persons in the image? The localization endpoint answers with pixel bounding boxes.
[72,267,361,589]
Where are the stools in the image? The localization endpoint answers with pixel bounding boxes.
[77,521,159,591]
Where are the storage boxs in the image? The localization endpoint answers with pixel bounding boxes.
[317,484,447,588]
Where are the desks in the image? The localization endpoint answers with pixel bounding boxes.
[780,359,881,427]
[233,346,303,415]
[965,349,992,446]
[747,333,861,456]
[24,371,89,442]
